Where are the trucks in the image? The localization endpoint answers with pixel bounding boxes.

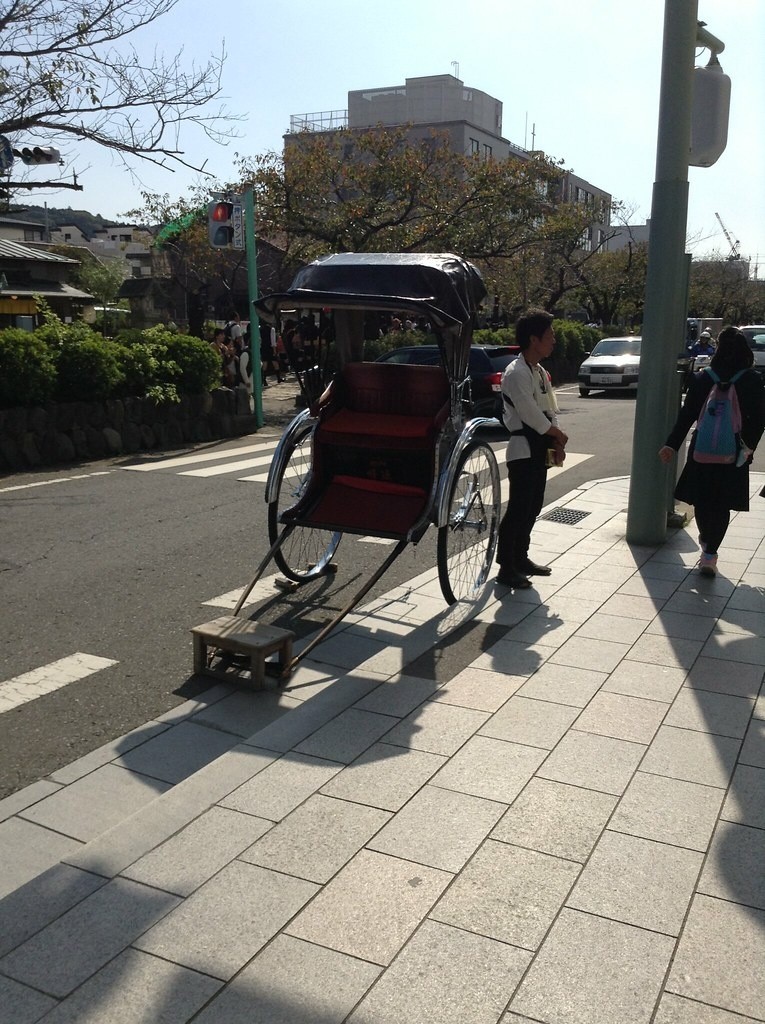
[686,316,723,348]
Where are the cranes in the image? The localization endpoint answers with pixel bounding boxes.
[714,211,742,261]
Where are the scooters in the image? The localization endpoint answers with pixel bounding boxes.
[687,345,712,389]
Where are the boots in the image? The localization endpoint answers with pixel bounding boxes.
[275,369,285,383]
[261,370,268,386]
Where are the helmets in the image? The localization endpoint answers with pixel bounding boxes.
[704,327,711,332]
[700,332,710,339]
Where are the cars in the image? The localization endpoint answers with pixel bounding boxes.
[577,335,642,397]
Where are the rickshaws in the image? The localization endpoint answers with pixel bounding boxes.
[202,250,509,695]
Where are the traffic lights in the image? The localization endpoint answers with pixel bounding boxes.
[207,200,235,250]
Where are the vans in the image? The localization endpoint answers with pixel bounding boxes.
[740,325,765,378]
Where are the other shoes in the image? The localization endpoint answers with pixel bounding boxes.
[517,557,552,575]
[495,566,533,588]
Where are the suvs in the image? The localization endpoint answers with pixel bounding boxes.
[369,343,553,430]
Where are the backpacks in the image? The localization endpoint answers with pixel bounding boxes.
[222,321,238,346]
[693,365,748,463]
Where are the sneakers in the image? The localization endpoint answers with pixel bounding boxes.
[695,534,718,575]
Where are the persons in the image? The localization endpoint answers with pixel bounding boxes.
[496,309,568,589]
[209,312,330,396]
[658,326,765,574]
[688,326,716,361]
[382,315,432,335]
[481,317,509,330]
